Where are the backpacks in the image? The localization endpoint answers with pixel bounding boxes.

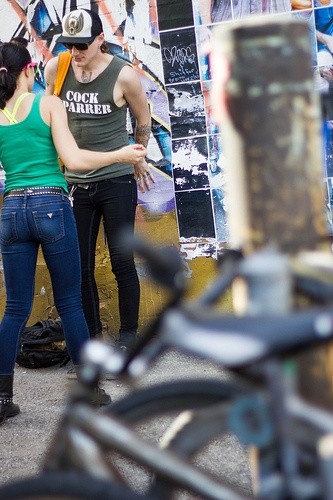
[16,319,71,369]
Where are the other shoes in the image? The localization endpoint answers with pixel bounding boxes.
[113,339,141,370]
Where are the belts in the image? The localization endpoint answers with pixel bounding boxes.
[3,187,69,200]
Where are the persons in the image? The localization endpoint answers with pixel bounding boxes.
[0,41,148,426]
[289,0,333,126]
[44,8,156,381]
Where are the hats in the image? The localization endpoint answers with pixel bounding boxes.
[55,8,103,44]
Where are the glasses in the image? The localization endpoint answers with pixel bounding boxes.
[22,62,38,72]
[62,35,96,50]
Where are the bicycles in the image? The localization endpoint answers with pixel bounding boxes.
[0,235,333,499]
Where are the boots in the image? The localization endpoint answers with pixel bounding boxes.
[0,374,20,422]
[76,365,113,408]
